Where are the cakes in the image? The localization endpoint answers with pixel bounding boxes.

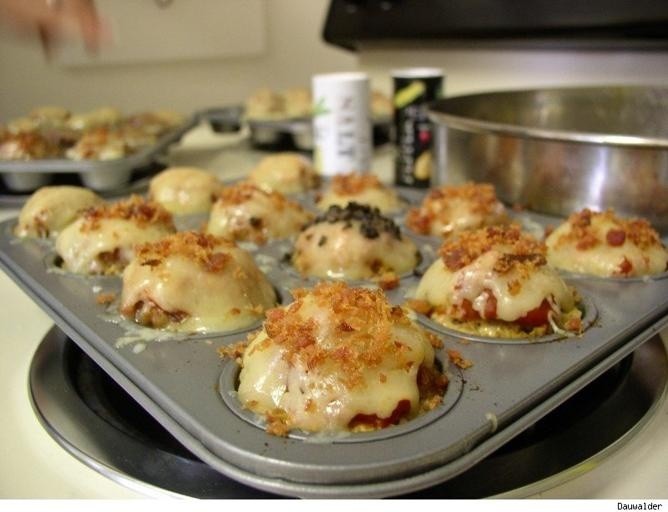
[1,107,173,160]
[245,81,313,120]
[371,91,394,118]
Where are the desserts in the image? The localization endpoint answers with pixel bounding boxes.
[15,152,668,440]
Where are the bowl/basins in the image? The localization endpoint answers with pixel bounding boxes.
[428,84,667,227]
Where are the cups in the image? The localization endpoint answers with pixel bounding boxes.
[310,67,446,190]
